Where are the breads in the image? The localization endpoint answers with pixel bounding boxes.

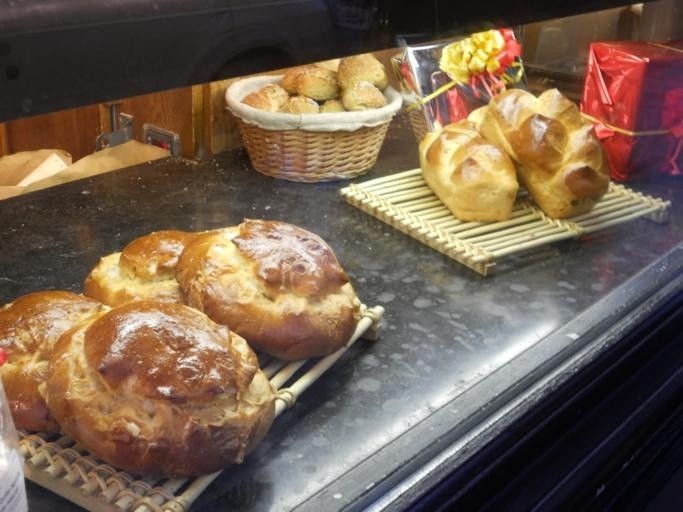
[2,291,109,429]
[282,67,304,92]
[262,82,290,108]
[338,54,388,91]
[85,230,197,303]
[344,82,386,111]
[296,67,337,99]
[478,87,609,216]
[417,123,520,221]
[243,93,275,114]
[278,98,319,118]
[174,218,357,358]
[36,299,277,473]
[322,102,346,114]
